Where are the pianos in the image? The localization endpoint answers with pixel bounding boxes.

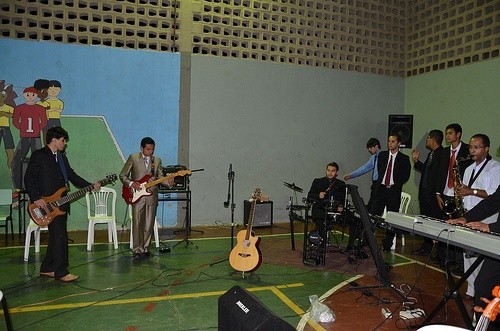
[383,211,500,330]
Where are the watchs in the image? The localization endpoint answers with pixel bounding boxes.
[472,189,477,195]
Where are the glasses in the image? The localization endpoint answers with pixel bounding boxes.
[467,146,486,150]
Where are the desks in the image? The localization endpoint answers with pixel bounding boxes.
[158,190,204,249]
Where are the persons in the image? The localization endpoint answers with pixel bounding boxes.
[410,130,444,256]
[369,133,411,250]
[308,161,361,254]
[120,137,174,256]
[462,133,500,299]
[24,126,101,282]
[446,185,500,331]
[344,138,383,210]
[436,123,469,272]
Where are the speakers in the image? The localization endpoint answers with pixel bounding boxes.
[244,199,274,228]
[217,284,297,331]
[388,113,413,148]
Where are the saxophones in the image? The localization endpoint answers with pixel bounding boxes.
[442,154,474,219]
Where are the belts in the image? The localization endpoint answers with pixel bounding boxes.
[380,184,393,188]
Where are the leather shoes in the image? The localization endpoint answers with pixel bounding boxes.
[412,248,431,255]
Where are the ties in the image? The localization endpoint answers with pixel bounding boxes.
[385,155,393,187]
[448,149,457,188]
[373,154,377,174]
[144,157,148,169]
[428,151,435,165]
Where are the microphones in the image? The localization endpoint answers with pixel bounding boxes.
[284,181,304,191]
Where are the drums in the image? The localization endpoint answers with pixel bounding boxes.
[287,204,310,210]
[308,230,324,245]
[323,200,340,205]
[302,198,320,204]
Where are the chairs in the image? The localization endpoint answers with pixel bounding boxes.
[23,219,50,262]
[383,192,410,251]
[129,204,159,248]
[86,187,118,250]
[0,189,14,246]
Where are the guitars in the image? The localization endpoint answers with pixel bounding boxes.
[228,188,263,273]
[474,285,500,331]
[28,173,118,226]
[122,167,192,205]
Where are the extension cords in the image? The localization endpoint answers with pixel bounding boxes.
[382,307,393,319]
[400,308,425,319]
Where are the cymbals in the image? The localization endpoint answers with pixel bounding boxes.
[335,184,358,194]
[283,181,303,193]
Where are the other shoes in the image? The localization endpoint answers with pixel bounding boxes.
[361,239,368,247]
[464,295,473,300]
[346,244,362,250]
[39,271,55,277]
[56,273,79,282]
[145,252,153,257]
[383,246,390,252]
[134,251,141,259]
